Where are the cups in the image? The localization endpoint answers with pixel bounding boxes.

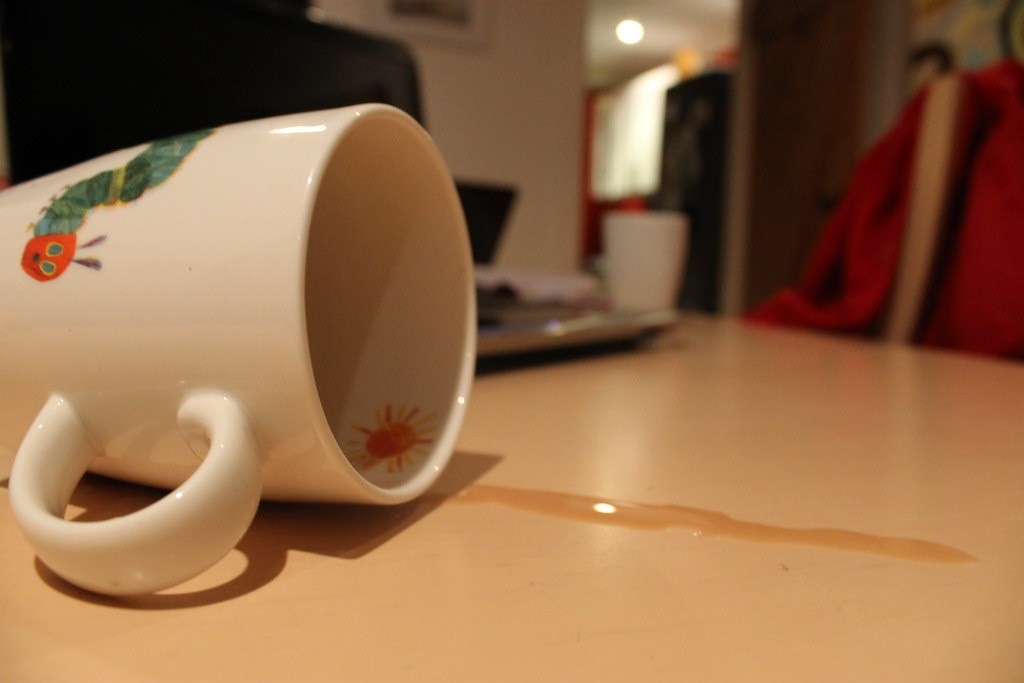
[0,102,477,598]
[606,210,689,312]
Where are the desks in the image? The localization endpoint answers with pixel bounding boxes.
[0,312,1024,683]
